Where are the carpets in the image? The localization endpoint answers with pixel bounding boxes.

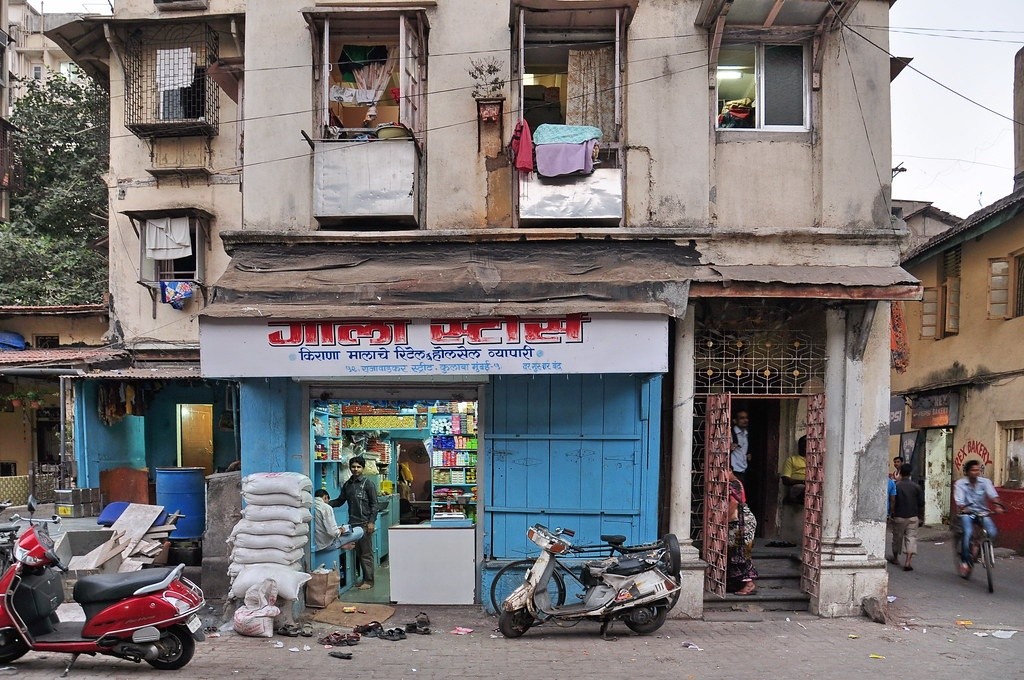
[313,601,395,629]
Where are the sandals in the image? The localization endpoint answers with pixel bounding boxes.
[300,623,313,636]
[318,633,345,646]
[735,585,755,595]
[278,624,300,637]
[343,633,359,645]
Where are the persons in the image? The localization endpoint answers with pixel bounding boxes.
[728,465,759,597]
[890,456,912,483]
[780,432,808,501]
[886,475,897,526]
[328,456,379,590]
[313,490,364,552]
[730,408,752,483]
[952,460,1002,575]
[890,462,925,571]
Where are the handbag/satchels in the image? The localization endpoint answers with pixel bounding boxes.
[306,560,340,608]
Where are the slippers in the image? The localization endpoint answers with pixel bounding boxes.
[765,541,776,547]
[377,629,399,640]
[394,628,406,639]
[776,540,797,547]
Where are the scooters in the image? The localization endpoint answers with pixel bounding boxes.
[0,498,21,576]
[499,523,683,642]
[0,494,207,678]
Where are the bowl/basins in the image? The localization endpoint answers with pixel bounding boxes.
[376,125,407,139]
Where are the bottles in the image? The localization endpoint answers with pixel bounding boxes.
[321,478,326,491]
[433,436,469,450]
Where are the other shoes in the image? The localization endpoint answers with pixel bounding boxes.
[972,544,979,556]
[406,612,431,634]
[890,557,900,565]
[353,621,383,636]
[904,566,913,571]
[961,564,968,577]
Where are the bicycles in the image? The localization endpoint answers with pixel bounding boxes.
[948,499,1009,593]
[9,513,62,528]
[490,526,682,628]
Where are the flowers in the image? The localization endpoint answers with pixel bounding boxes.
[390,88,400,103]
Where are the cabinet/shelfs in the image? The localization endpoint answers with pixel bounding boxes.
[310,400,480,597]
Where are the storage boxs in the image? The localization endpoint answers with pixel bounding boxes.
[343,105,399,128]
[50,530,122,602]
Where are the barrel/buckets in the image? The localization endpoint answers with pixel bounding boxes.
[157,467,206,539]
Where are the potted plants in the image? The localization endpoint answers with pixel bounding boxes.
[464,57,510,121]
[8,391,26,407]
[27,390,46,408]
